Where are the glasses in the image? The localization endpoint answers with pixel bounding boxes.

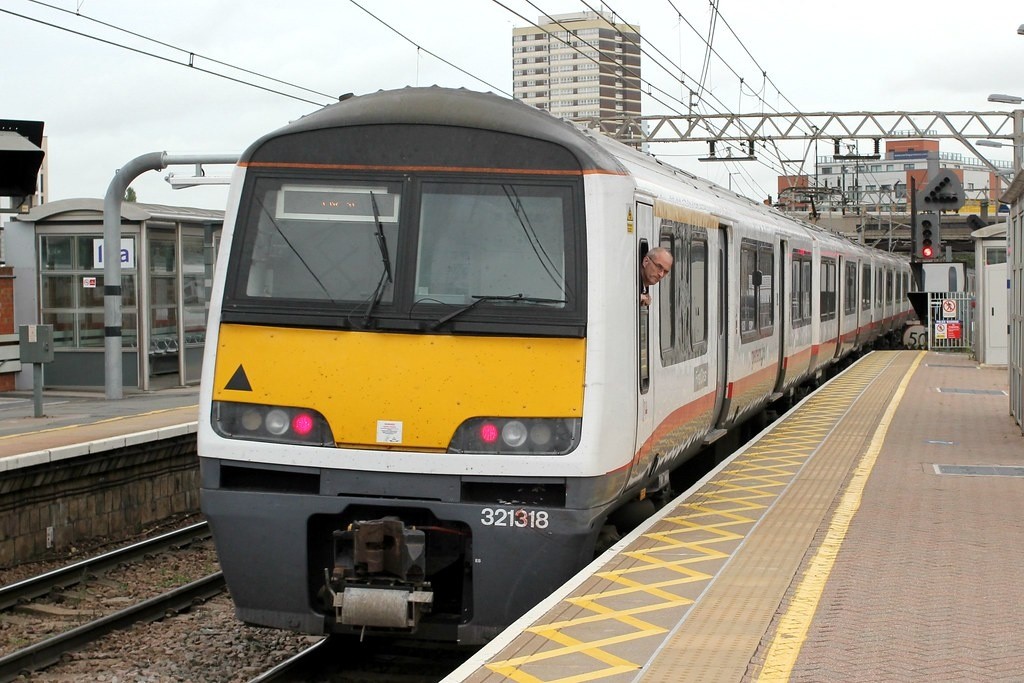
[648,256,669,275]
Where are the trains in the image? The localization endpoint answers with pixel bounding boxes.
[198,82,975,653]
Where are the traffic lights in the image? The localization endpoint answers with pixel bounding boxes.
[913,214,940,259]
[918,168,966,212]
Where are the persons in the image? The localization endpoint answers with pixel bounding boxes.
[639,247,673,306]
